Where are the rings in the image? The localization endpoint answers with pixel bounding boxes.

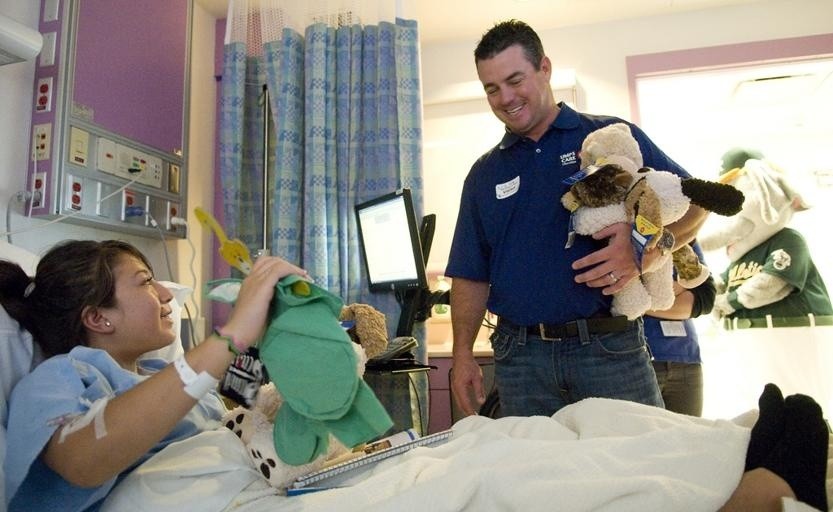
[610,273,617,282]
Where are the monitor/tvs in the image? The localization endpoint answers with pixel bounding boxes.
[354,188,428,293]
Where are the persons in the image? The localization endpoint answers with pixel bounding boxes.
[445,19,719,418]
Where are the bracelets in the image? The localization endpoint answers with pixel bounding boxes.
[213,325,249,356]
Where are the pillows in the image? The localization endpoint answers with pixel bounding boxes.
[6,265,192,408]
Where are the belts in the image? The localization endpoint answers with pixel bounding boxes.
[496,315,629,341]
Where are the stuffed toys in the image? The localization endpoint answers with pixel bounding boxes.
[696,146,833,327]
[572,122,745,320]
[223,303,387,488]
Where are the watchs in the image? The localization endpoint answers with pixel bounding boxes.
[656,227,675,256]
